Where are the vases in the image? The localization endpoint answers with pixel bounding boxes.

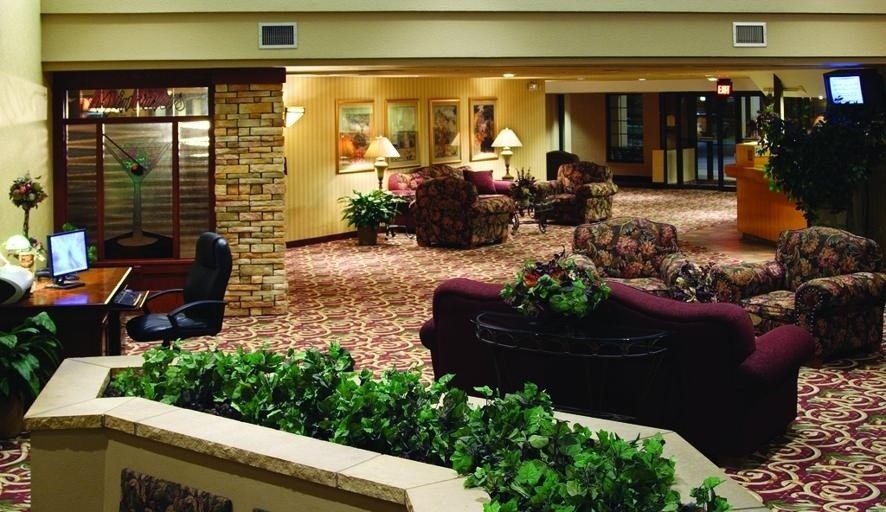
[22,210,29,240]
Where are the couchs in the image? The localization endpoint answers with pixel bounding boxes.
[419,279,812,463]
[572,215,711,306]
[703,227,886,362]
[388,164,514,231]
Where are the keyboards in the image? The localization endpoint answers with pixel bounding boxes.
[113,286,143,307]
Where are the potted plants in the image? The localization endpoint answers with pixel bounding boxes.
[0,311,67,438]
[23,334,770,511]
[336,188,408,246]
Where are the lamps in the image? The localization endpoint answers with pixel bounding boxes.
[363,135,401,192]
[284,106,305,129]
[491,127,523,179]
[716,78,733,97]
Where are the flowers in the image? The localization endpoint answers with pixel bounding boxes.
[498,244,613,325]
[8,169,48,237]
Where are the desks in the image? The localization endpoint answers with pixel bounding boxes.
[461,313,672,423]
[0,265,149,415]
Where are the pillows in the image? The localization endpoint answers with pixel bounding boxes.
[463,169,497,194]
[401,171,425,190]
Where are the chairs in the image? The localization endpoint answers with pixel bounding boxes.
[125,232,232,350]
[415,176,515,248]
[535,160,619,226]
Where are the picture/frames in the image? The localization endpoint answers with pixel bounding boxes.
[383,98,421,170]
[335,98,376,174]
[428,97,463,165]
[467,96,499,162]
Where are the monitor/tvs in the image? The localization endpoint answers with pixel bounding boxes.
[823,70,875,109]
[46,228,90,289]
